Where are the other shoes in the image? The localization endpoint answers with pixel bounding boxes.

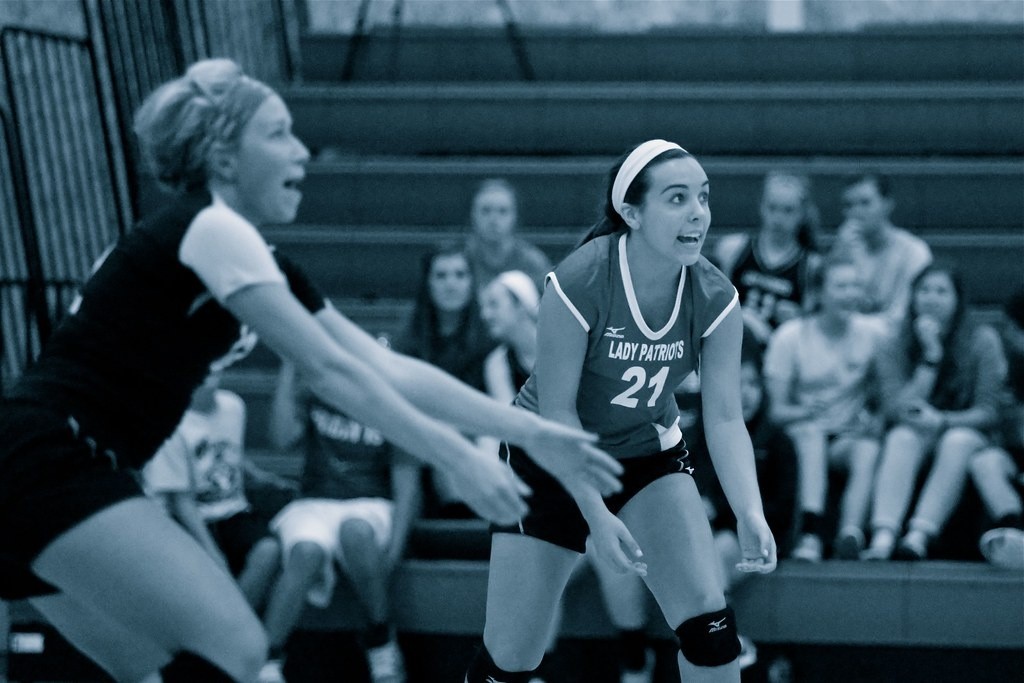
[866,533,928,560]
[258,660,283,683]
[792,534,823,562]
[979,528,1024,572]
[833,525,864,561]
[369,627,404,683]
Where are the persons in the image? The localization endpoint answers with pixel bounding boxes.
[476,269,654,683]
[261,352,420,683]
[399,242,498,395]
[1,58,623,682]
[140,366,280,611]
[678,169,1024,568]
[465,138,778,683]
[453,179,553,292]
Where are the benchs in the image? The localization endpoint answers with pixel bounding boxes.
[210,22,1024,646]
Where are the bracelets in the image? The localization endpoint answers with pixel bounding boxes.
[920,359,940,367]
